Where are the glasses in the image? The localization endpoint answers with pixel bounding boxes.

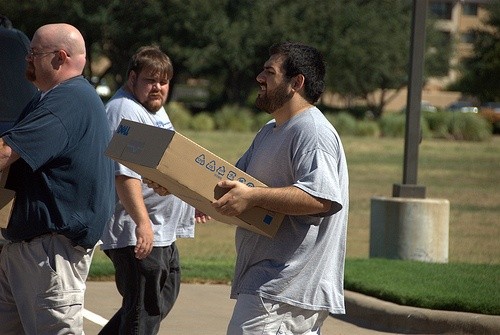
[28,49,70,60]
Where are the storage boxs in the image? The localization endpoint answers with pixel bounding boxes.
[103,119,287,239]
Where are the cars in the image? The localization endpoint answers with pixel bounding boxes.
[400,99,499,135]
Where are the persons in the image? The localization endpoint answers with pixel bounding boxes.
[0,23,115,335]
[0,15,40,138]
[98,46,211,335]
[142,41,349,335]
[89,44,122,104]
